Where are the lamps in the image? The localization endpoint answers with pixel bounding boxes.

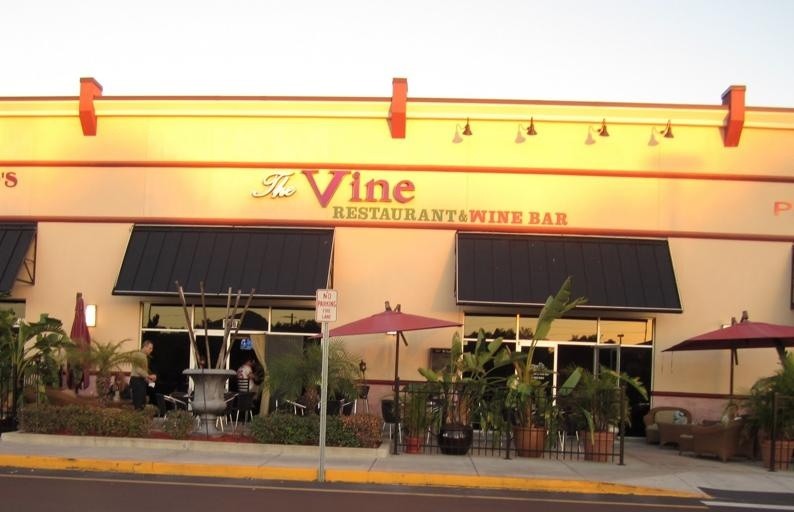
[463,118,472,136]
[527,117,537,136]
[598,119,609,137]
[660,120,674,138]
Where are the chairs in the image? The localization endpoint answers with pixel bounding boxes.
[643,404,760,463]
[110,377,369,432]
[380,399,402,444]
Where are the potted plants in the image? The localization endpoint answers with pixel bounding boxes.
[495,277,586,456]
[419,326,504,455]
[571,361,647,462]
[721,351,794,470]
[394,385,438,453]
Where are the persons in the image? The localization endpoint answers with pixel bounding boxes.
[129,340,156,411]
[236,358,260,401]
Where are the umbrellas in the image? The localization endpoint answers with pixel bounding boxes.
[661,310,794,402]
[66,292,91,394]
[307,300,465,398]
